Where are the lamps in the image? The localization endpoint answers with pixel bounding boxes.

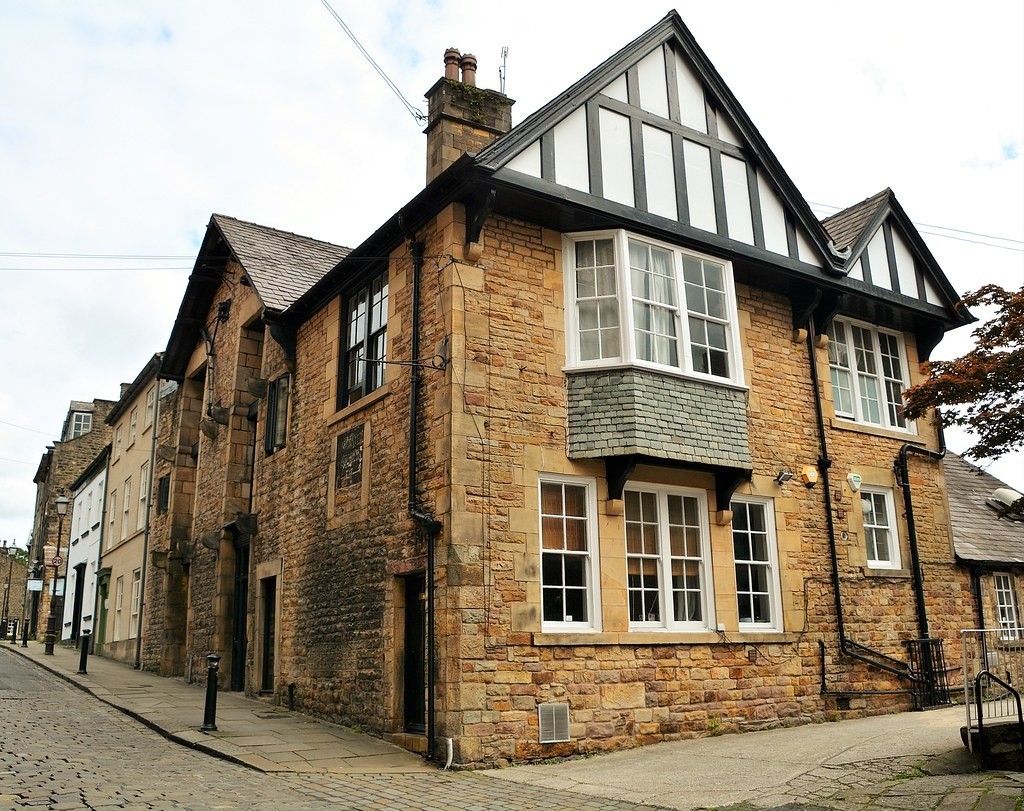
[774,472,794,486]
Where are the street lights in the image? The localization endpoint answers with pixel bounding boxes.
[44,485,70,656]
[3,538,19,641]
[0,576,10,638]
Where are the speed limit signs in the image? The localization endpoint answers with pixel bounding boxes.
[51,555,64,567]
